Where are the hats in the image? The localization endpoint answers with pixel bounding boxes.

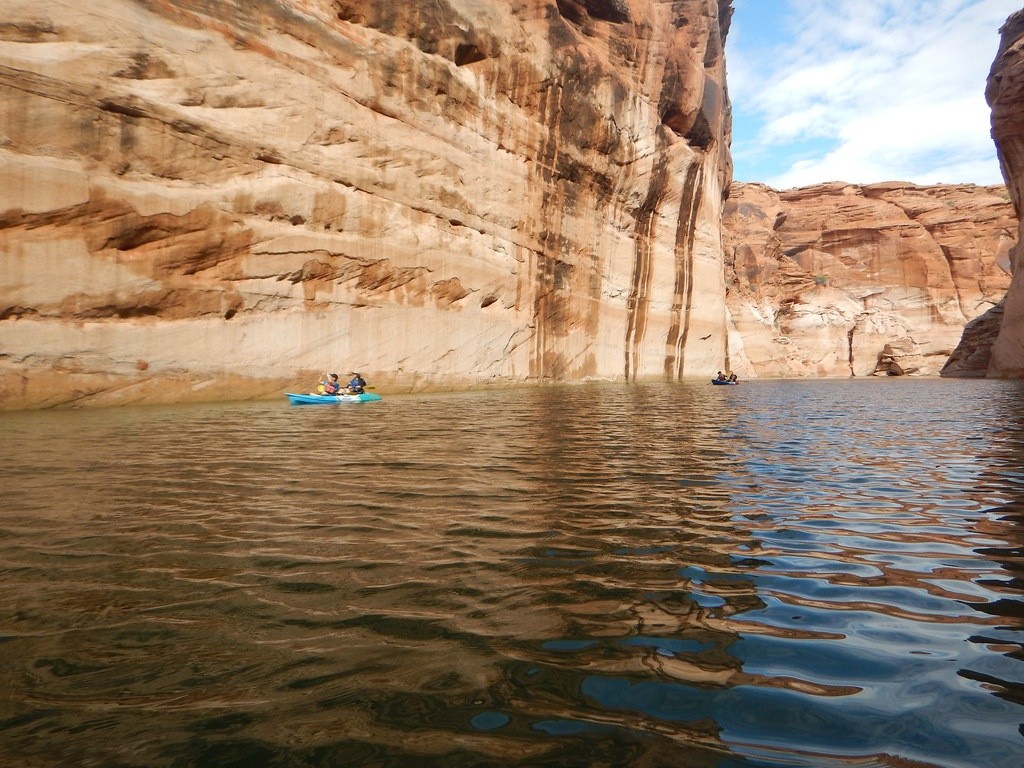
[329,373,338,379]
[352,370,360,374]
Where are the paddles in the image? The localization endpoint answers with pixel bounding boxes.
[318,385,375,392]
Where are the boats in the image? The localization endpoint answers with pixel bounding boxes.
[711,379,740,386]
[284,392,384,405]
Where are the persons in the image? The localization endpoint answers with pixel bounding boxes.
[716,371,738,382]
[318,371,366,395]
